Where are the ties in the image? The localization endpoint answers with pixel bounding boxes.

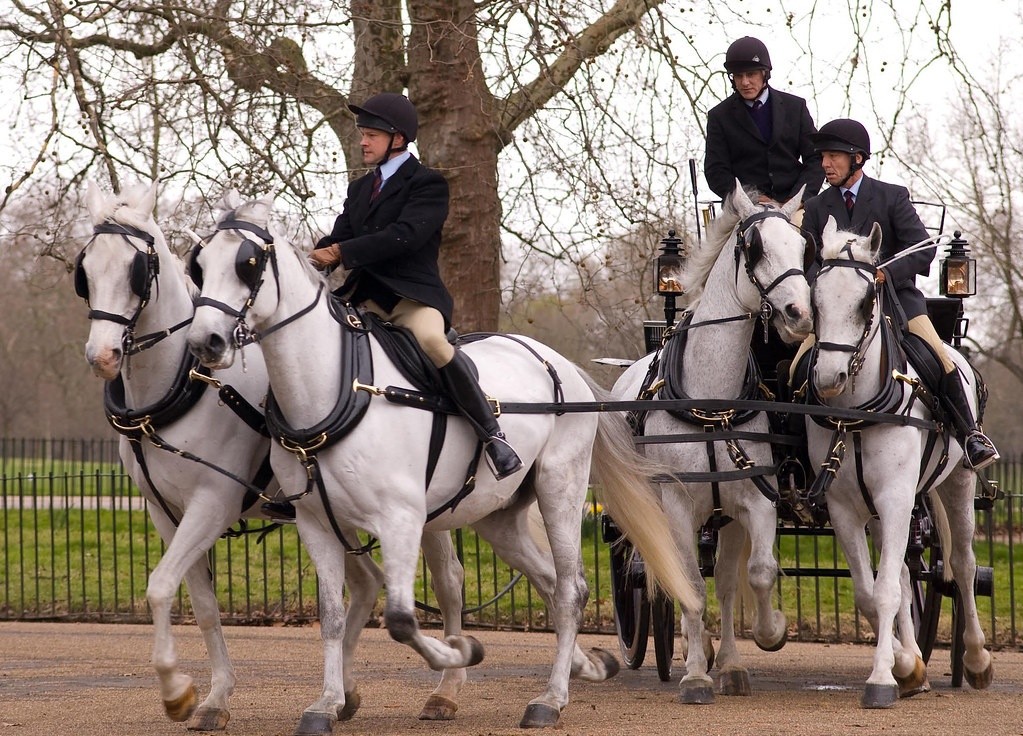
[844,191,855,222]
[369,167,383,202]
[752,100,761,110]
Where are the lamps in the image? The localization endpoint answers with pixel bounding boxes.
[939,229,976,298]
[650,230,688,297]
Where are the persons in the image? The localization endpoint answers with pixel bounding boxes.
[770,119,997,469]
[261,92,523,520]
[705,35,823,219]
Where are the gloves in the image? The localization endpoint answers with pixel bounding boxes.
[306,243,342,270]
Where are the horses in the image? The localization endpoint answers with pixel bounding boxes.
[609,177,813,705]
[812,215,993,709]
[81,179,466,733]
[184,180,704,733]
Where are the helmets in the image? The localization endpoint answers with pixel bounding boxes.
[724,36,772,72]
[348,92,419,143]
[808,117,871,161]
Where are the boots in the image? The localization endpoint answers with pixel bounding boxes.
[437,345,521,476]
[937,361,995,465]
[783,384,812,487]
[261,500,296,521]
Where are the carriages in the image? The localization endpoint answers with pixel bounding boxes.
[74,159,1007,736]
